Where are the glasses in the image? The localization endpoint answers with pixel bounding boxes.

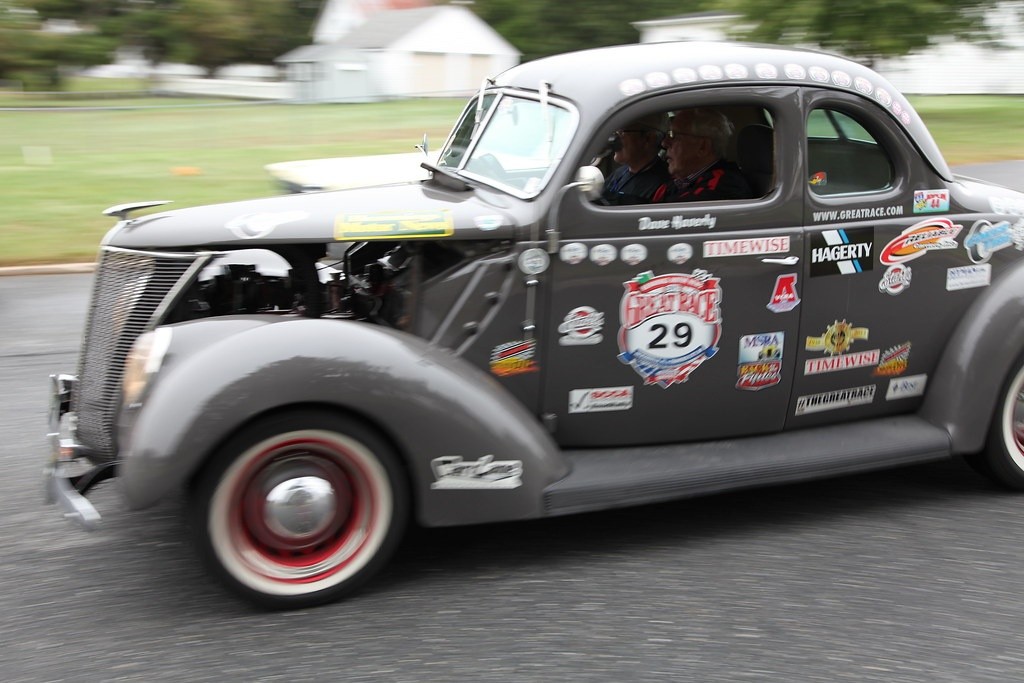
[666,129,711,141]
[612,129,648,136]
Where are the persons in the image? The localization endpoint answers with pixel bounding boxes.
[606,111,671,198]
[602,108,749,206]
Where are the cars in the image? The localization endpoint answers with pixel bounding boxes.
[41,43,1024,611]
